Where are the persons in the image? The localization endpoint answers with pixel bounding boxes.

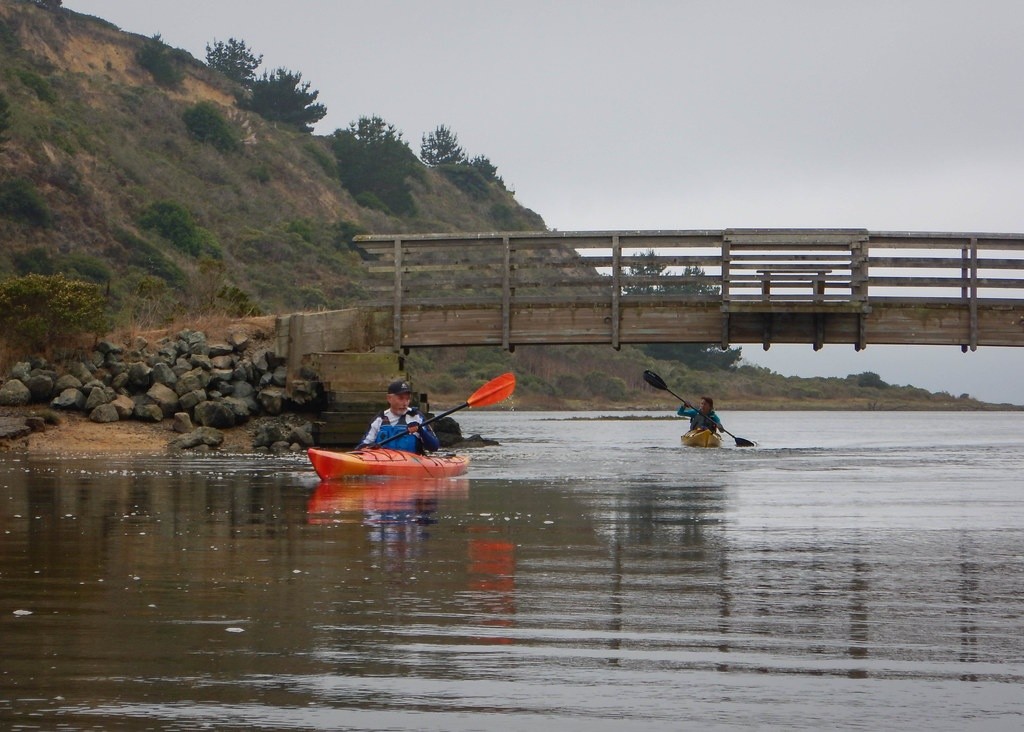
[362,381,439,457]
[677,397,725,436]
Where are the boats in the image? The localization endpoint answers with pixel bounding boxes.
[680,427,722,448]
[307,477,469,524]
[308,448,470,479]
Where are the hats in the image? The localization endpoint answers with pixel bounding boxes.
[701,397,713,407]
[388,381,412,395]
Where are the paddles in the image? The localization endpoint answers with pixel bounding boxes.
[367,372,517,449]
[641,369,753,446]
